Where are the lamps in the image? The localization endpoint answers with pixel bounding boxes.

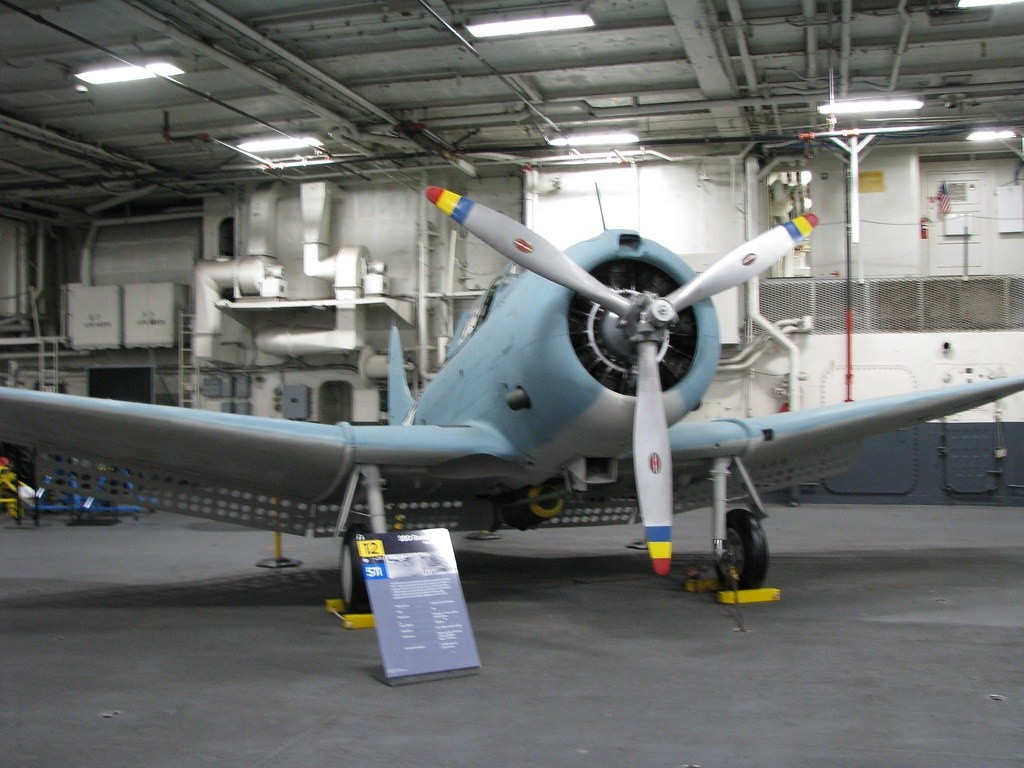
[74,55,185,85]
[236,132,327,152]
[816,96,927,114]
[548,126,640,146]
[463,4,596,37]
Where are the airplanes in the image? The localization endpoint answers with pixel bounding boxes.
[0,176,1023,591]
